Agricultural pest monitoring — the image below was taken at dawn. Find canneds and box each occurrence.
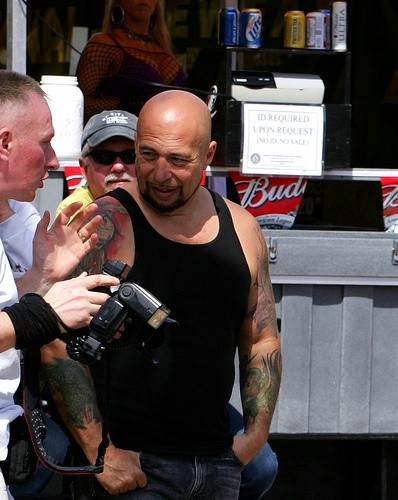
[240,8,262,48]
[282,10,327,50]
[217,7,240,46]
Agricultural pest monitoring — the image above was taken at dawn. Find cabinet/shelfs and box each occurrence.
[220,43,352,171]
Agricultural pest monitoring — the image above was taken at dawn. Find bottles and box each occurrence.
[38,75,84,160]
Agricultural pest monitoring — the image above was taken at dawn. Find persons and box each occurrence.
[75,1,187,124]
[0,70,120,500]
[25,110,280,500]
[46,90,283,500]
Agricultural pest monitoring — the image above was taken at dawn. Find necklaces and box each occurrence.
[119,23,156,43]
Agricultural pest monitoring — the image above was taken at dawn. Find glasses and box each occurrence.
[84,149,136,165]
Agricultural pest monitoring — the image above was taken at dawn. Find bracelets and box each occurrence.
[3,292,61,351]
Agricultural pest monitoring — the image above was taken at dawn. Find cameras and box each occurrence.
[66,259,170,363]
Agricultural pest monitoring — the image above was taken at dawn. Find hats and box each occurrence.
[81,109,138,150]
